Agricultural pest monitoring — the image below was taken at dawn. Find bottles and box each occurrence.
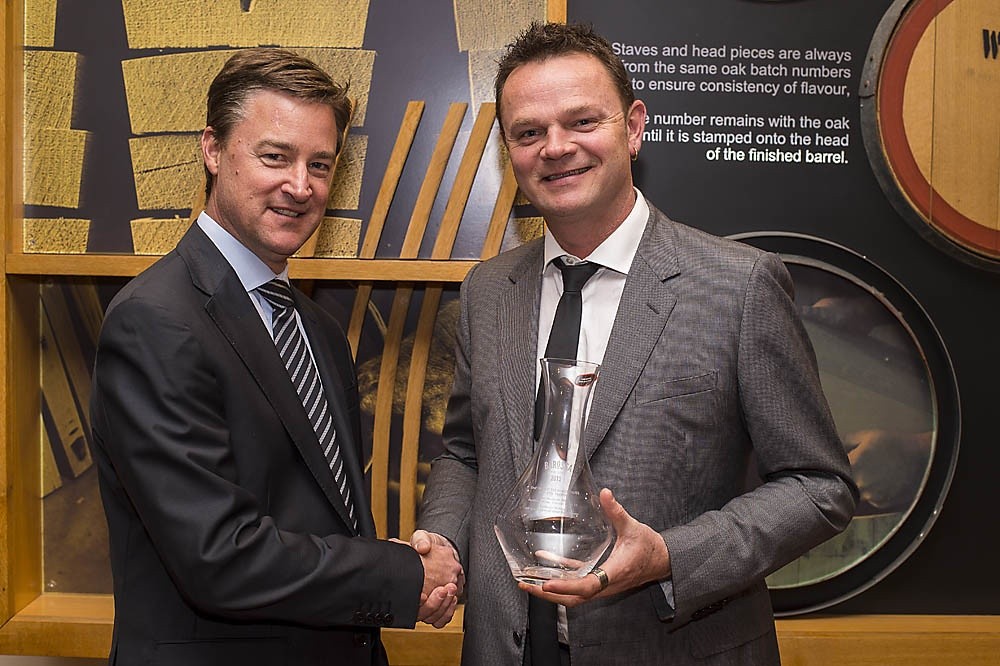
[493,358,613,589]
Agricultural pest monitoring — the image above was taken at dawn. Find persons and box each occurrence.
[90,45,464,666]
[411,20,865,666]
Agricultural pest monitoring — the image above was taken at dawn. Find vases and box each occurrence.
[493,357,613,586]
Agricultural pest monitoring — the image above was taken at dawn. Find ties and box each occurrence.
[255,279,360,539]
[526,256,602,666]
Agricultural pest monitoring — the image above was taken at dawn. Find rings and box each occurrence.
[591,569,609,591]
